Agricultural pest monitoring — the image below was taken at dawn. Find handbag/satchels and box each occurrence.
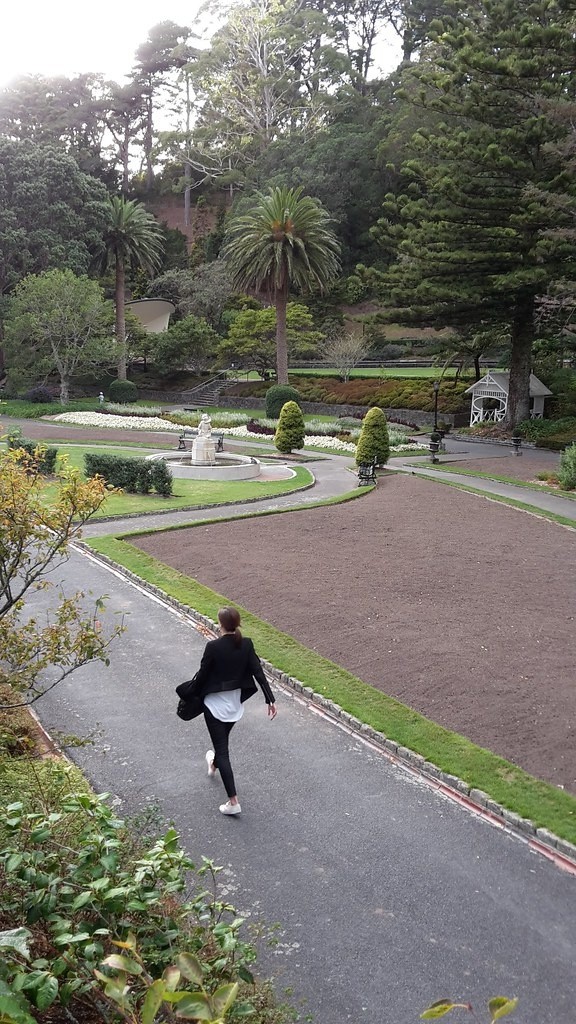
[177,671,203,721]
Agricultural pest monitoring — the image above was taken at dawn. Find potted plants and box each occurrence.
[428,433,441,449]
[510,428,523,443]
[435,421,445,436]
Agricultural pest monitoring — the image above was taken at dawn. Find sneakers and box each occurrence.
[219,801,241,814]
[206,750,216,777]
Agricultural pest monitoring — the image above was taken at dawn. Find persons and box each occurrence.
[218,436,223,451]
[197,606,277,815]
[198,414,212,440]
[98,392,104,404]
[179,434,185,447]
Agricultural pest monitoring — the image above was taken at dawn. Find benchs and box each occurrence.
[178,428,224,452]
[358,456,379,488]
